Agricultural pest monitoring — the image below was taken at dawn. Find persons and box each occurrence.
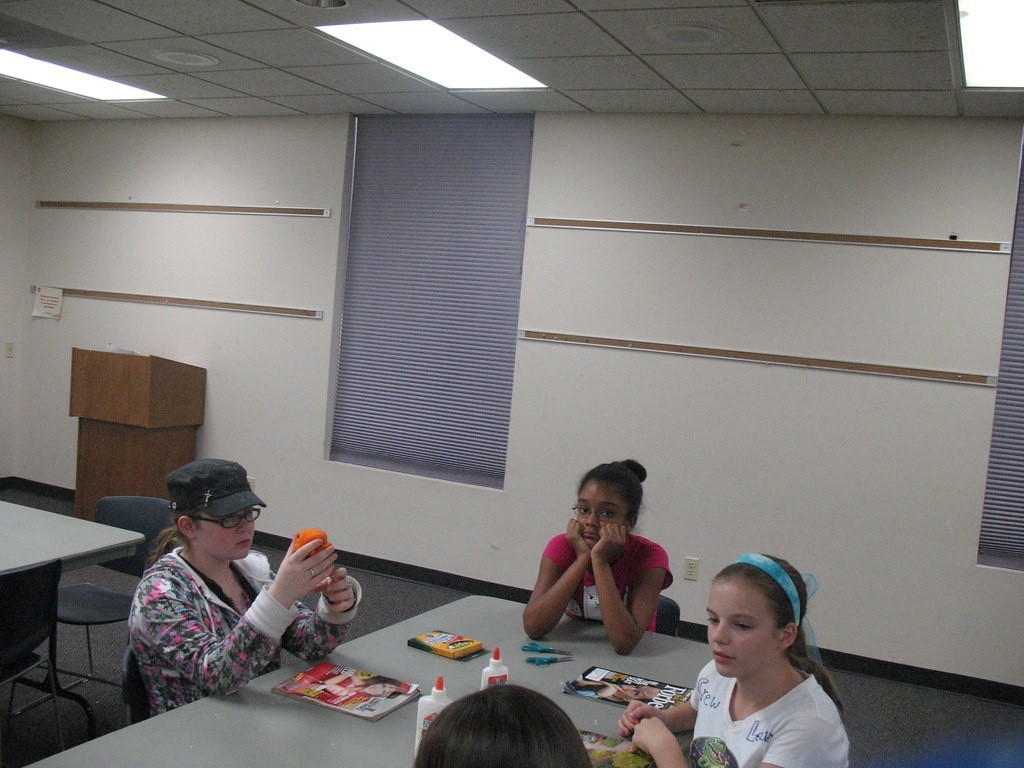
[523,459,673,656]
[412,686,593,768]
[128,459,363,718]
[617,553,849,768]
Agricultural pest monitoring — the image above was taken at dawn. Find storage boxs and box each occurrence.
[407,630,483,659]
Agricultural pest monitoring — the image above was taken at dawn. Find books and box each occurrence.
[271,660,422,722]
[562,665,694,711]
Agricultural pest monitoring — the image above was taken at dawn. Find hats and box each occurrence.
[166,458,267,516]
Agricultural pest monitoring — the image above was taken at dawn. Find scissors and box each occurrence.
[521,642,572,654]
[526,656,572,666]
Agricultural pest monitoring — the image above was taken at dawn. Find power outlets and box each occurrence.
[247,476,256,491]
[684,557,700,581]
[4,342,14,358]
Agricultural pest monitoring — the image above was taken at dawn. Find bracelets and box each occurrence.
[323,588,356,613]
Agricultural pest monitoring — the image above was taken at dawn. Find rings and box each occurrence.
[310,569,315,576]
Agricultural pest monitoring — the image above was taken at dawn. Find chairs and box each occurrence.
[0,559,63,754]
[54,495,172,683]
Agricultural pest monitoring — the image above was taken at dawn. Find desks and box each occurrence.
[0,500,145,741]
[19,595,715,768]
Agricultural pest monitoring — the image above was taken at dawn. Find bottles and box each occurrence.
[479,646,508,690]
[413,675,455,759]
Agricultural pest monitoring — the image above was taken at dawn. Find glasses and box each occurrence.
[571,506,629,522]
[186,508,261,528]
